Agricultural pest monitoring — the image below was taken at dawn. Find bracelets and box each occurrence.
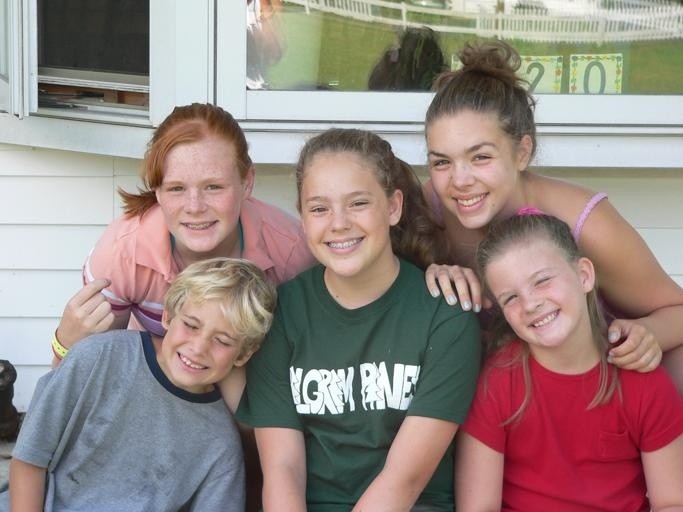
[50,344,63,363]
[51,326,68,359]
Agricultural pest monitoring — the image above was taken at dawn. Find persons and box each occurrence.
[231,125,487,512]
[0,255,280,511]
[453,205,682,512]
[50,101,319,511]
[417,33,682,396]
[363,23,445,93]
